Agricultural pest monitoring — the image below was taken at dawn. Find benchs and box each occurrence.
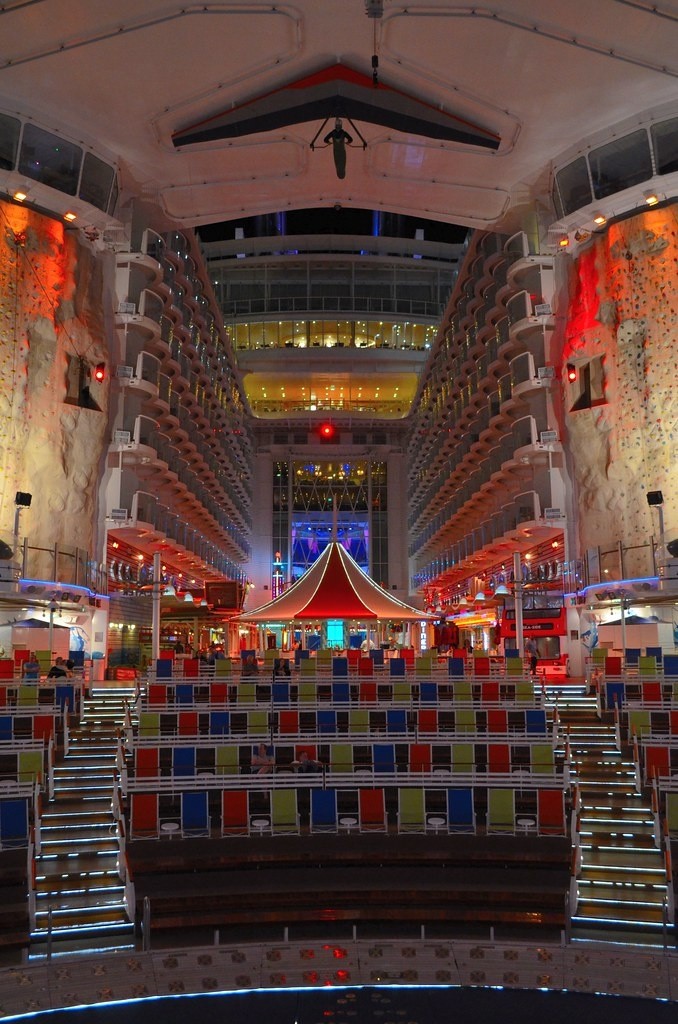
[0,835,571,956]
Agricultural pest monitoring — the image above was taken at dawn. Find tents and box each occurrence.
[224,542,444,650]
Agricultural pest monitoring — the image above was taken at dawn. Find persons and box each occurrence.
[525,634,541,674]
[251,744,276,799]
[447,639,474,656]
[23,654,41,686]
[275,658,291,676]
[239,633,249,661]
[361,636,376,651]
[47,657,74,679]
[292,639,302,651]
[140,654,150,666]
[243,655,260,676]
[291,752,323,773]
[175,640,226,678]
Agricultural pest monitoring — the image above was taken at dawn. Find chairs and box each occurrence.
[0,646,678,836]
